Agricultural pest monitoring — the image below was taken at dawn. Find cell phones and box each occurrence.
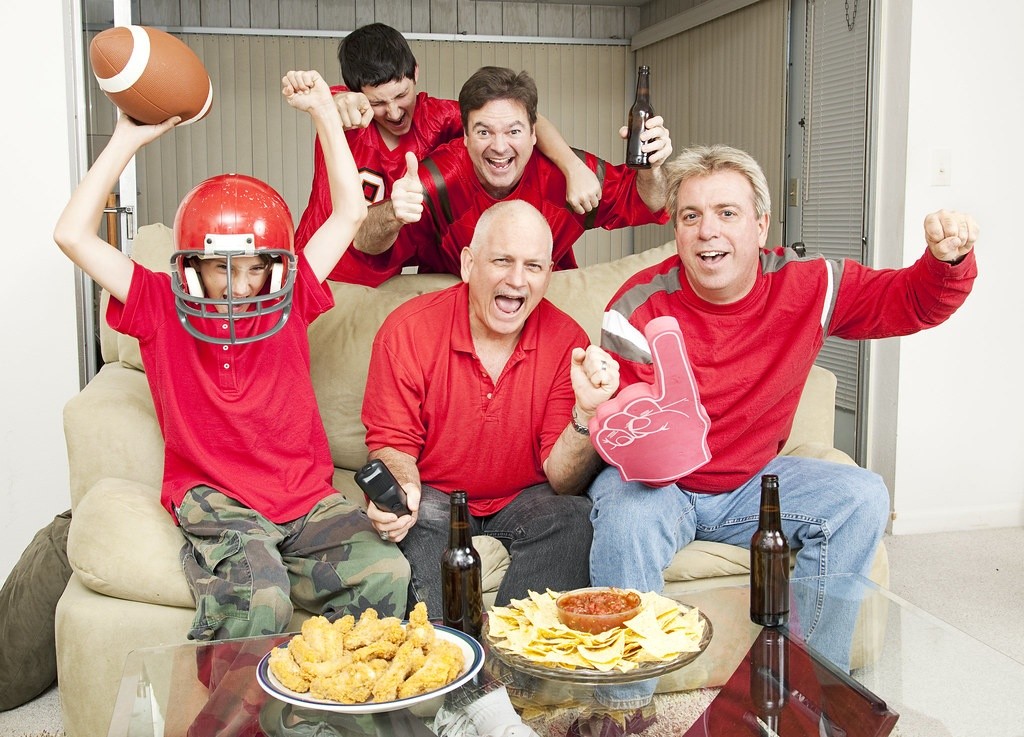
[354,458,412,517]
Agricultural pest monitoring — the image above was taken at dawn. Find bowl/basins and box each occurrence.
[556,587,643,636]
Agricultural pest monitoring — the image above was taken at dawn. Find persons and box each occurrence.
[281,23,602,288]
[563,143,977,737]
[51,64,416,736]
[358,198,622,737]
[352,55,674,285]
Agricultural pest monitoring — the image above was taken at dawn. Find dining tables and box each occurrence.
[111,570,1024,737]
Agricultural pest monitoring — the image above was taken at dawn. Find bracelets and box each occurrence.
[569,404,594,437]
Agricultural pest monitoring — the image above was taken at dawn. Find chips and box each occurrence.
[487,587,705,671]
[507,692,663,729]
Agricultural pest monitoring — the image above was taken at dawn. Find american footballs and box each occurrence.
[89,24,214,128]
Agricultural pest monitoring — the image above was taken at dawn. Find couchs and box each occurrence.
[54,222,889,737]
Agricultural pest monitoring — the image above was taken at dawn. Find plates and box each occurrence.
[256,618,486,715]
[480,590,715,685]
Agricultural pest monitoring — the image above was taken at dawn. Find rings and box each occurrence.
[379,531,389,541]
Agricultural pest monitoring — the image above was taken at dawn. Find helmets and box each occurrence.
[176,173,296,296]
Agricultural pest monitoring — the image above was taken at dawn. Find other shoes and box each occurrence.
[566,696,656,737]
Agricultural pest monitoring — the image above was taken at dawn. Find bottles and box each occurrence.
[625,65,655,169]
[749,474,791,627]
[749,625,789,737]
[441,490,484,646]
